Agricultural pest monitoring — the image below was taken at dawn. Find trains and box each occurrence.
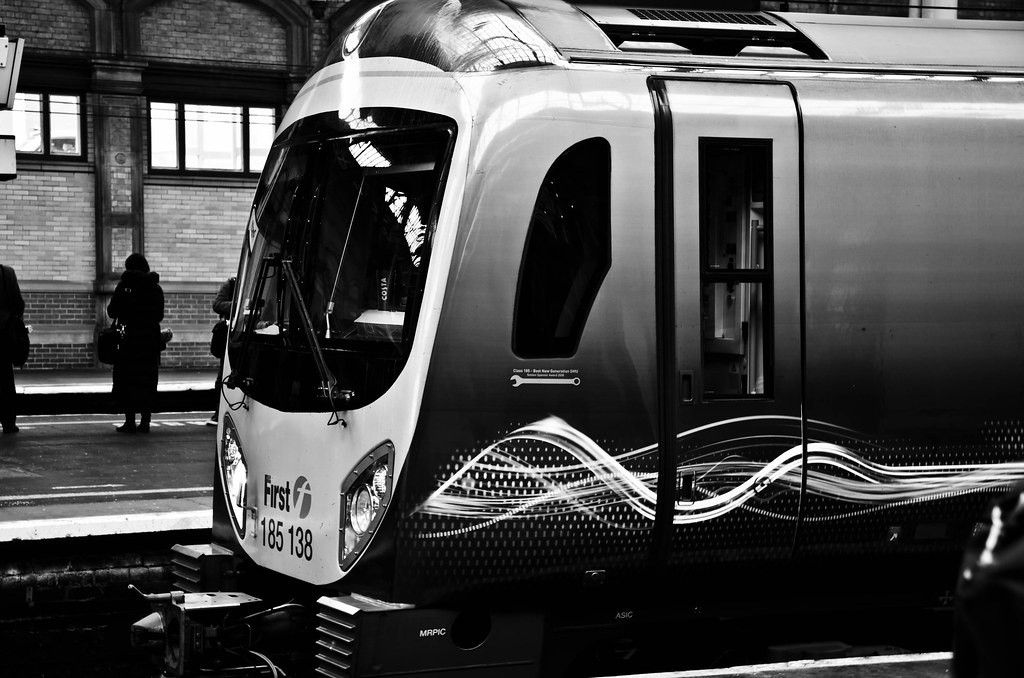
[127,1,1024,678]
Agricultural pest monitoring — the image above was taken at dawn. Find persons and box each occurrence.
[0,263,30,436]
[206,276,237,425]
[106,253,164,431]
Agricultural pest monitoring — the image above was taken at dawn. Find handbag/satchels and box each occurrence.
[210,320,228,357]
[98,317,123,365]
[5,317,30,366]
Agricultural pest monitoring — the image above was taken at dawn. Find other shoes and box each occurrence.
[116,420,136,433]
[3,424,19,434]
[207,413,218,426]
[137,420,149,432]
